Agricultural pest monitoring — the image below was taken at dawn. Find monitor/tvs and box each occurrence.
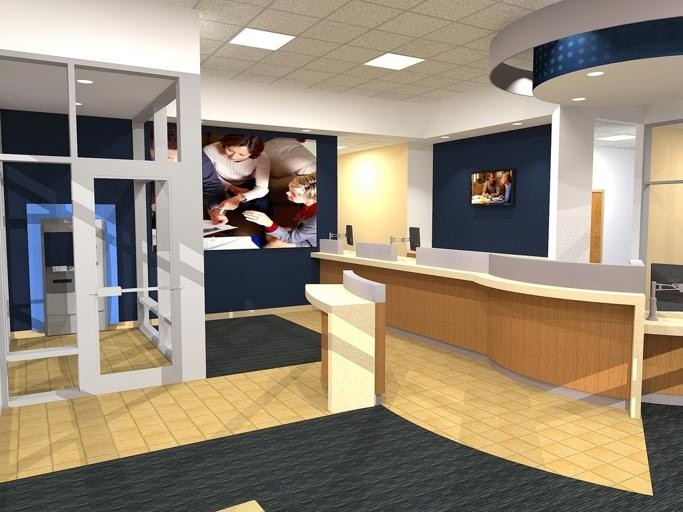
[410,227,420,251]
[470,169,515,206]
[347,225,353,245]
[651,263,683,311]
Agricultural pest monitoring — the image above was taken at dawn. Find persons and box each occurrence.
[242,172,318,249]
[203,133,272,233]
[149,127,229,226]
[490,172,512,203]
[482,172,500,198]
